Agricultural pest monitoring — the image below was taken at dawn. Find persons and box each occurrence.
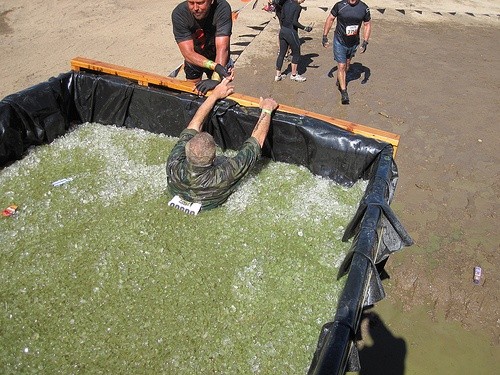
[322,0,372,104]
[165,76,279,216]
[261,0,315,82]
[171,0,235,95]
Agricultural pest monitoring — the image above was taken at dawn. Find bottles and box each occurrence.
[473,266,481,284]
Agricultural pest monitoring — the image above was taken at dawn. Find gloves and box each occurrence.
[304,26,312,32]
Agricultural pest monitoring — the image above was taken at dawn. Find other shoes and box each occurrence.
[341,89,349,103]
[290,73,307,82]
[336,78,347,92]
[274,74,286,82]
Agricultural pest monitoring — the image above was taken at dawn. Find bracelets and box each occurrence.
[206,60,213,69]
[262,108,272,115]
